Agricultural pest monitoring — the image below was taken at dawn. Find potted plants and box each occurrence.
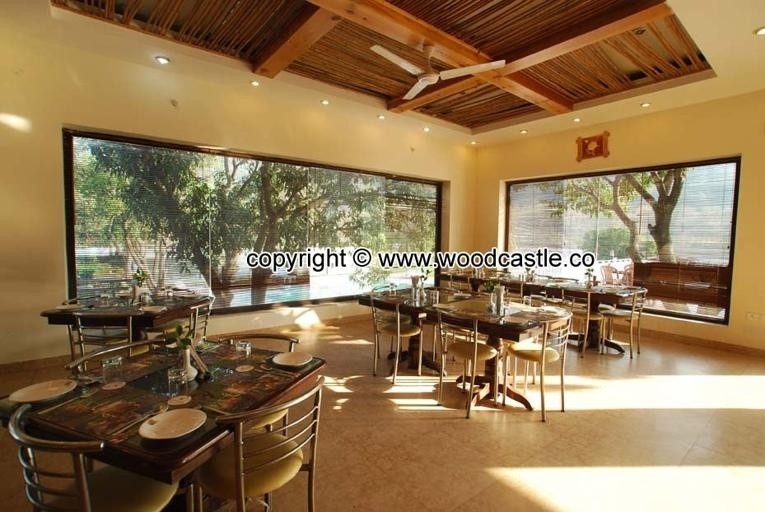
[130,266,151,304]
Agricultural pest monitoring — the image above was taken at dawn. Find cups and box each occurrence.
[523,295,531,312]
[235,342,251,370]
[100,355,124,390]
[430,290,438,306]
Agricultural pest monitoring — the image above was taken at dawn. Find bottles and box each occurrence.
[168,367,188,406]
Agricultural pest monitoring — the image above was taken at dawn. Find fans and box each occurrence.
[368,43,506,102]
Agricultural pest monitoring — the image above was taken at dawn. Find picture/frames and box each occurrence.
[576,131,610,162]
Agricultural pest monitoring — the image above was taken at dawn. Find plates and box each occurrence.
[138,306,167,314]
[272,351,313,367]
[8,378,77,404]
[55,304,86,312]
[138,409,208,440]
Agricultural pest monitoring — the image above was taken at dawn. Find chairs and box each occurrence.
[359,261,647,413]
[0,286,327,512]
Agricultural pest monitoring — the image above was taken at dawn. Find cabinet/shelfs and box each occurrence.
[717,267,730,309]
[632,263,679,298]
[679,264,717,304]
